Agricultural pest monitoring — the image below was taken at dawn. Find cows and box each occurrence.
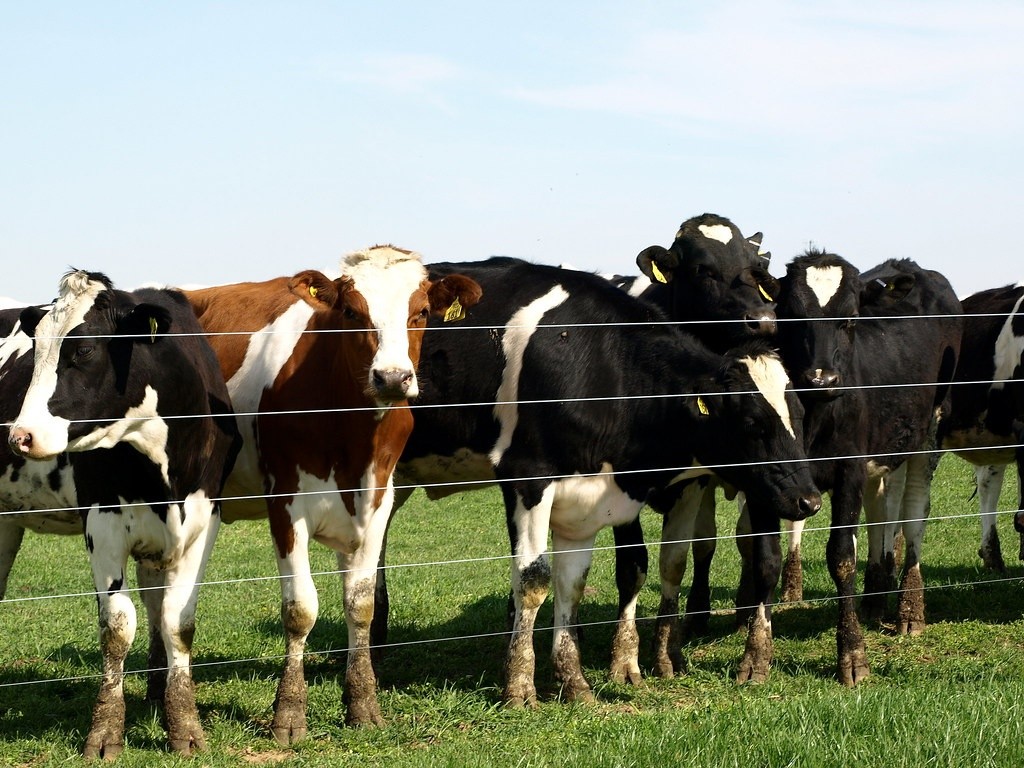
[0,213,1024,762]
